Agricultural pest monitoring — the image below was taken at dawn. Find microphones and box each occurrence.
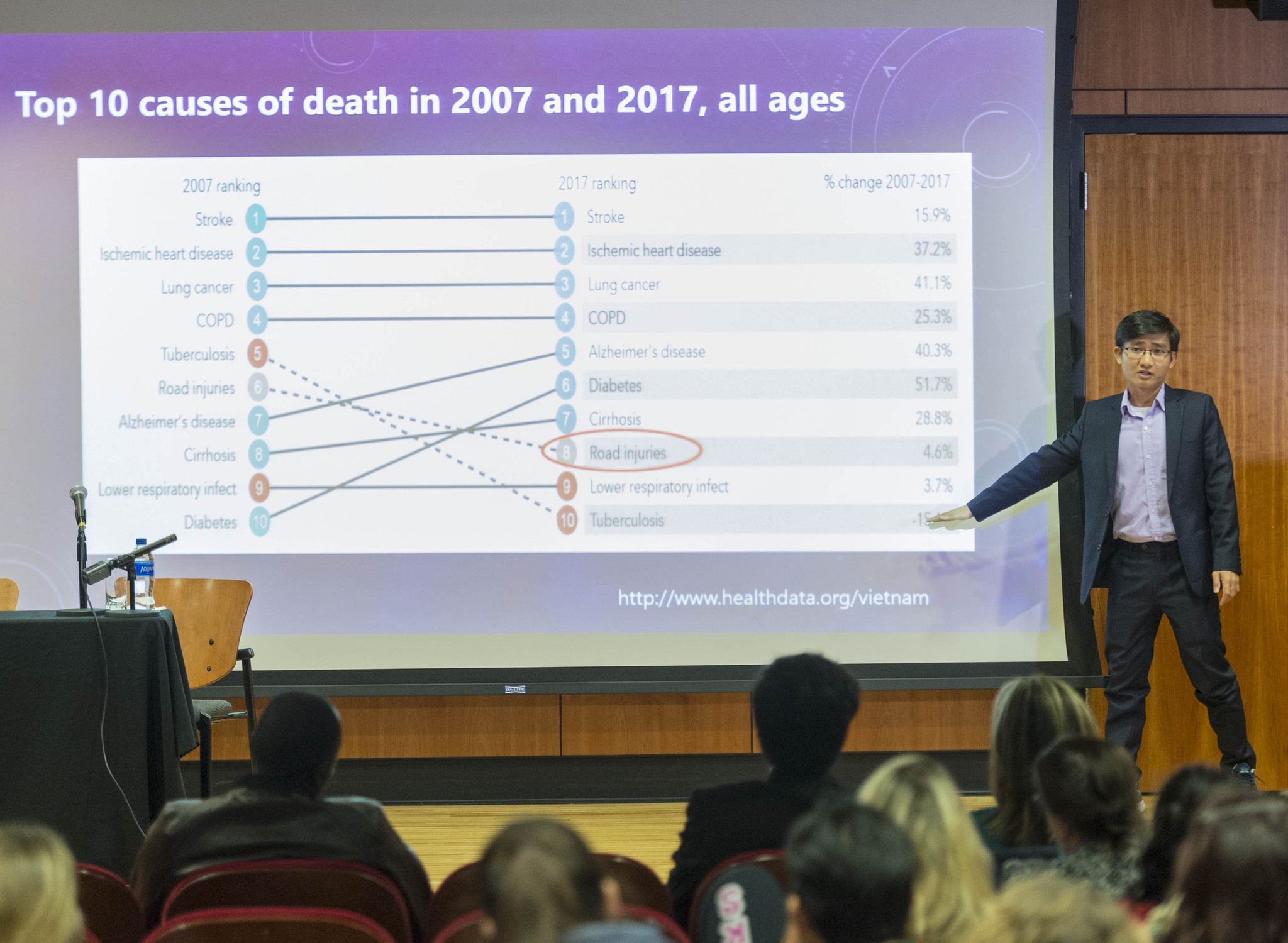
[82,534,177,586]
[70,485,87,532]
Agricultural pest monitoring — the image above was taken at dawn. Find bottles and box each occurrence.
[128,538,156,610]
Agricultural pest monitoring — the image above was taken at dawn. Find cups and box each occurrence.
[104,558,127,611]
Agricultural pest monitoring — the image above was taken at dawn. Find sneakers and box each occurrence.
[1230,763,1265,791]
[1135,783,1146,814]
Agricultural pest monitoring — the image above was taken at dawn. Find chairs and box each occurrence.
[114,578,257,800]
[71,853,794,943]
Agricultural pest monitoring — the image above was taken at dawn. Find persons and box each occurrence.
[0,652,1288,943]
[926,310,1256,815]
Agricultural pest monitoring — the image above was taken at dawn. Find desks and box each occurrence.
[0,607,202,894]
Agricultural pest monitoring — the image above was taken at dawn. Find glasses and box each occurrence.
[1120,346,1172,358]
